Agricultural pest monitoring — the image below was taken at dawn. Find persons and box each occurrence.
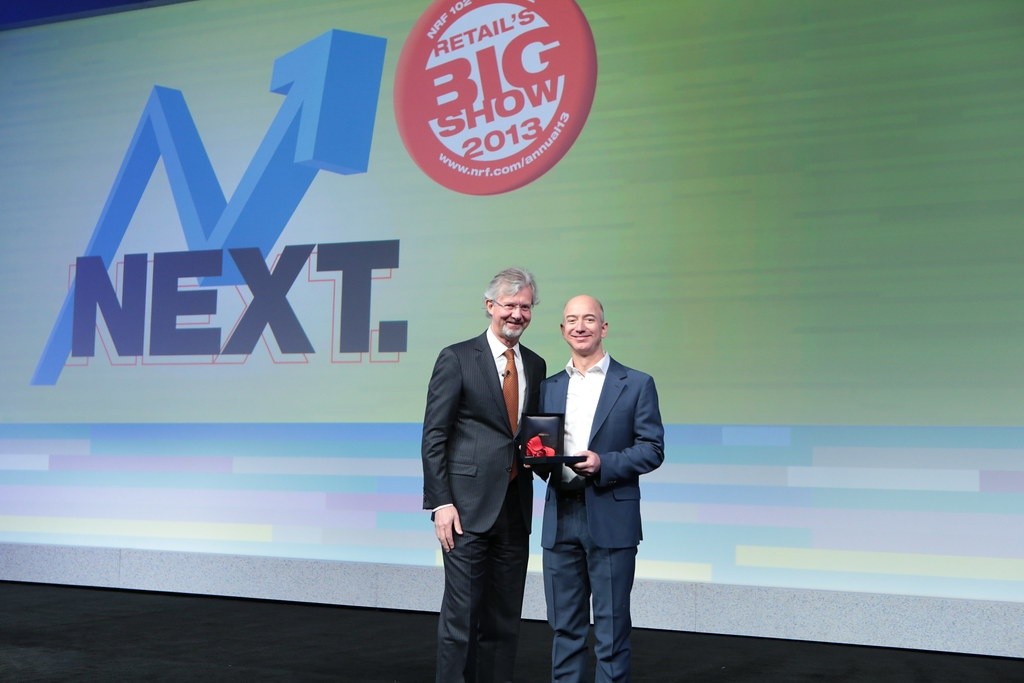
[518,294,665,683]
[421,267,547,683]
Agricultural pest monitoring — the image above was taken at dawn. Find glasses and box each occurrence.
[493,300,532,313]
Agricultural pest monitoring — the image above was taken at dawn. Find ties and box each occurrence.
[503,349,519,482]
